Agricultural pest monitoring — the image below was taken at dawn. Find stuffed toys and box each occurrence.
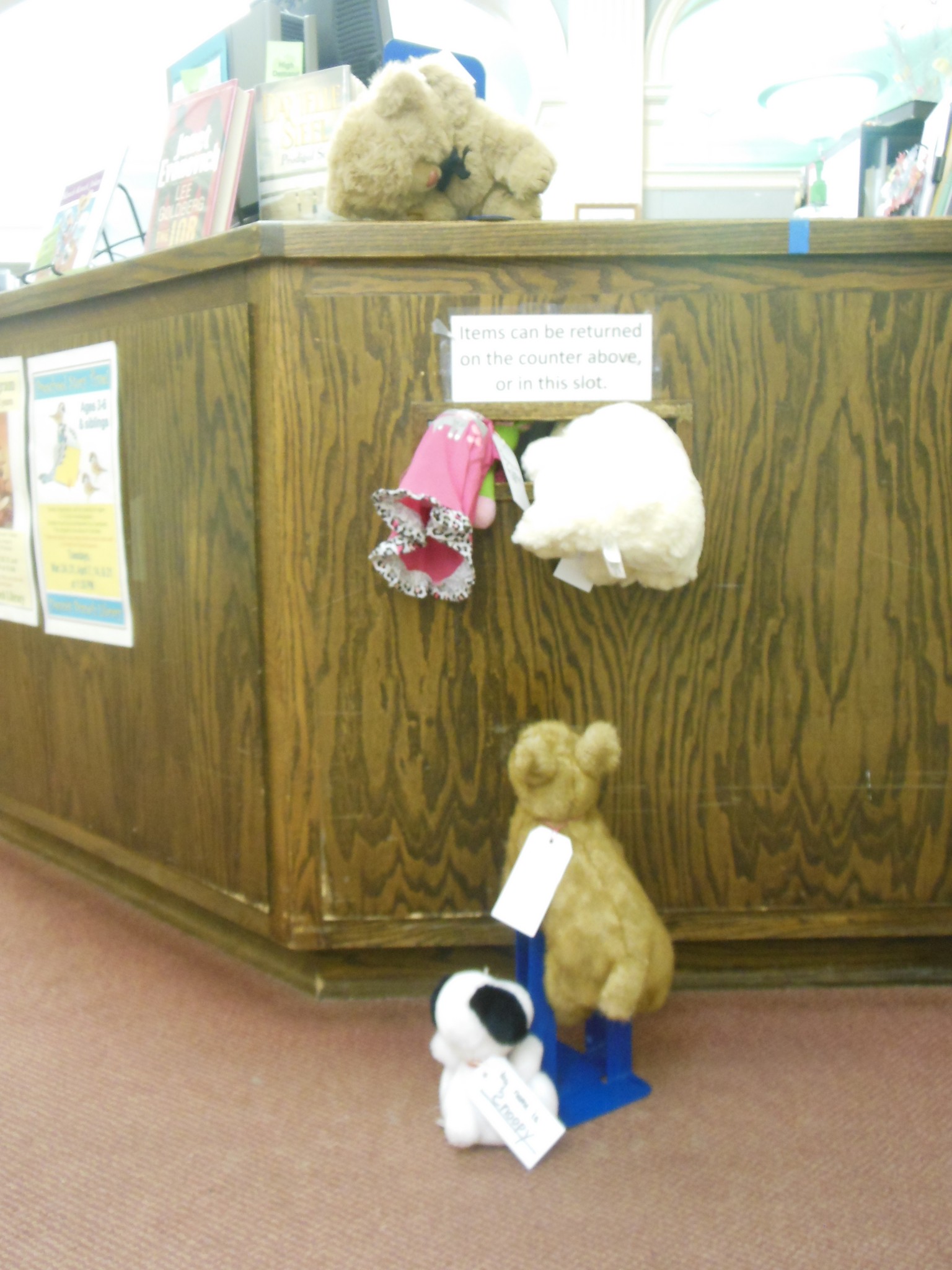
[428,967,559,1149]
[500,718,675,1028]
[326,48,556,222]
[366,400,706,604]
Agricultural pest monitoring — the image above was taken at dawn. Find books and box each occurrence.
[24,166,109,284]
[252,64,351,222]
[141,79,253,252]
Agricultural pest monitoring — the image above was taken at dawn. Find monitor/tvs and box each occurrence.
[164,0,393,225]
